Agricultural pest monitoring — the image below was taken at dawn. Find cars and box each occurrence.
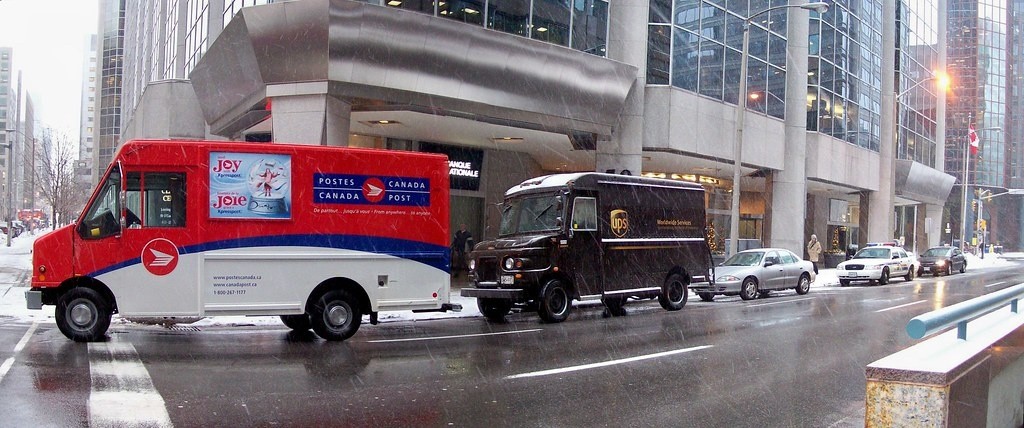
[837,242,915,287]
[0,216,48,240]
[917,246,968,277]
[690,247,816,301]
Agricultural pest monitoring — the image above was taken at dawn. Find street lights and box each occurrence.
[960,126,1002,251]
[729,2,830,256]
[889,70,951,248]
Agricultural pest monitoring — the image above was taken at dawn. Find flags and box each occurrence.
[969,123,980,154]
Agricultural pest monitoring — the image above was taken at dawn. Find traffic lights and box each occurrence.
[988,190,993,203]
[972,201,977,212]
[974,190,979,197]
[982,220,986,228]
[974,219,979,231]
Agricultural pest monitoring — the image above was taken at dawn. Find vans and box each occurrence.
[23,140,463,342]
[461,173,715,323]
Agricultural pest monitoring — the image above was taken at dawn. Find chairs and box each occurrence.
[156,184,181,226]
[579,220,595,229]
[870,250,877,256]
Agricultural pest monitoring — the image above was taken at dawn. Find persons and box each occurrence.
[807,234,821,275]
[454,223,474,279]
